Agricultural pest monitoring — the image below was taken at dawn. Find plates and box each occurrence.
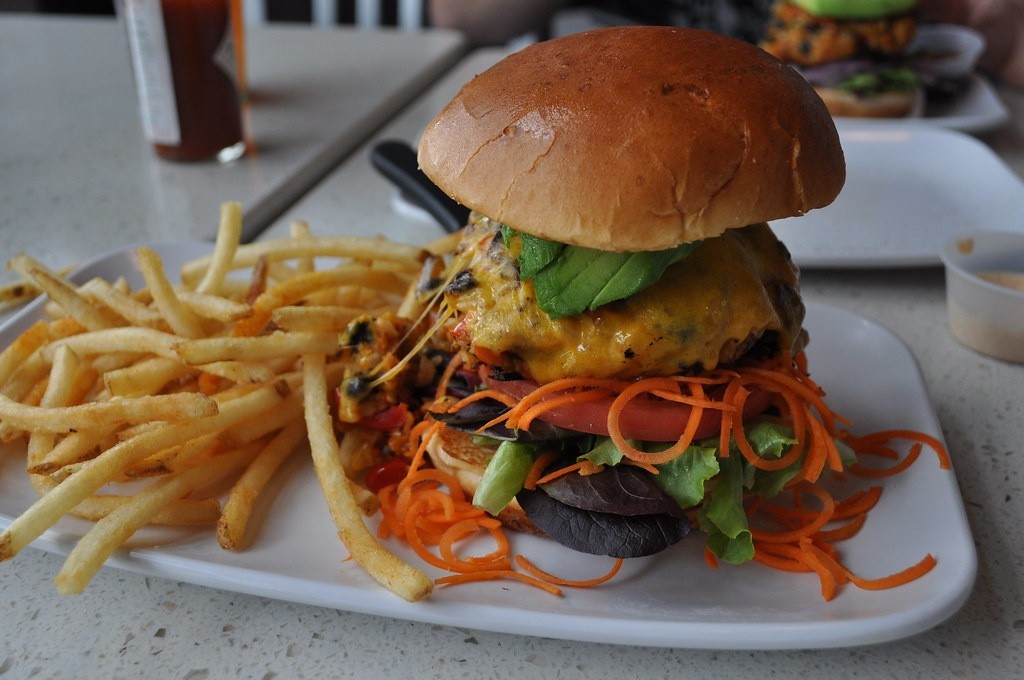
[767,126,1024,271]
[832,71,1009,135]
[1,239,979,651]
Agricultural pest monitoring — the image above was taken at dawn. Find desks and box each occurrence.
[0,13,1024,680]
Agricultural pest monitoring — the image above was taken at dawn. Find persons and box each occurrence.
[426,0,777,52]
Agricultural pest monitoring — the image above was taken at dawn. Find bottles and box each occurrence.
[114,0,257,164]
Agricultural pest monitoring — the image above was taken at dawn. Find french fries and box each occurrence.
[0,200,467,604]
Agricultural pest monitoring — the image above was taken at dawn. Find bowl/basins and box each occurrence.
[935,230,1024,364]
[903,25,985,79]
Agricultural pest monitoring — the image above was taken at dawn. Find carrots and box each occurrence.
[690,429,954,598]
[371,471,623,596]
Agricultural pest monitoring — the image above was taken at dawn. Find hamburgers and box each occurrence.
[340,25,848,561]
[752,1,932,120]
